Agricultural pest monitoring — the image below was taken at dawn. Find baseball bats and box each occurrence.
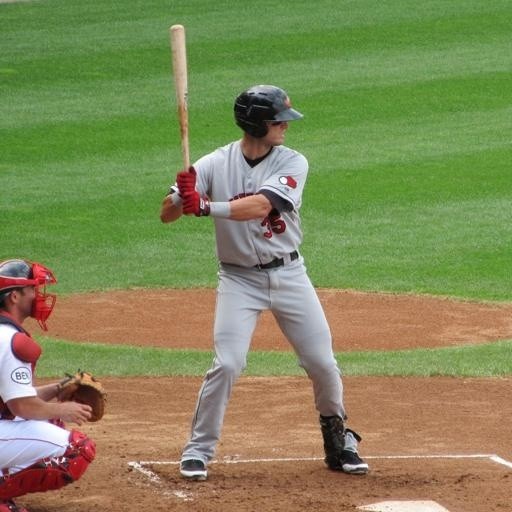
[170,24,192,215]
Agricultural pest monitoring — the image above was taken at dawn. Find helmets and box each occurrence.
[0,259,56,331]
[234,85,304,138]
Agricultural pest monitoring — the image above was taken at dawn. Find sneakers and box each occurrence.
[326,444,370,474]
[179,459,208,481]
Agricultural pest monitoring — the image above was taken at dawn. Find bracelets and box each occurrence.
[210,201,231,219]
[171,189,185,208]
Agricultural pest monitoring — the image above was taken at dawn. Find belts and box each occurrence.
[256,250,298,271]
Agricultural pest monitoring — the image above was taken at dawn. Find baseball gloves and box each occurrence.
[57,371,106,422]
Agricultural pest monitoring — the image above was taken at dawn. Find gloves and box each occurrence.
[175,165,210,217]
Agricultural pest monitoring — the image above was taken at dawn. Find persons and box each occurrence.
[160,84,369,483]
[0,258,96,512]
